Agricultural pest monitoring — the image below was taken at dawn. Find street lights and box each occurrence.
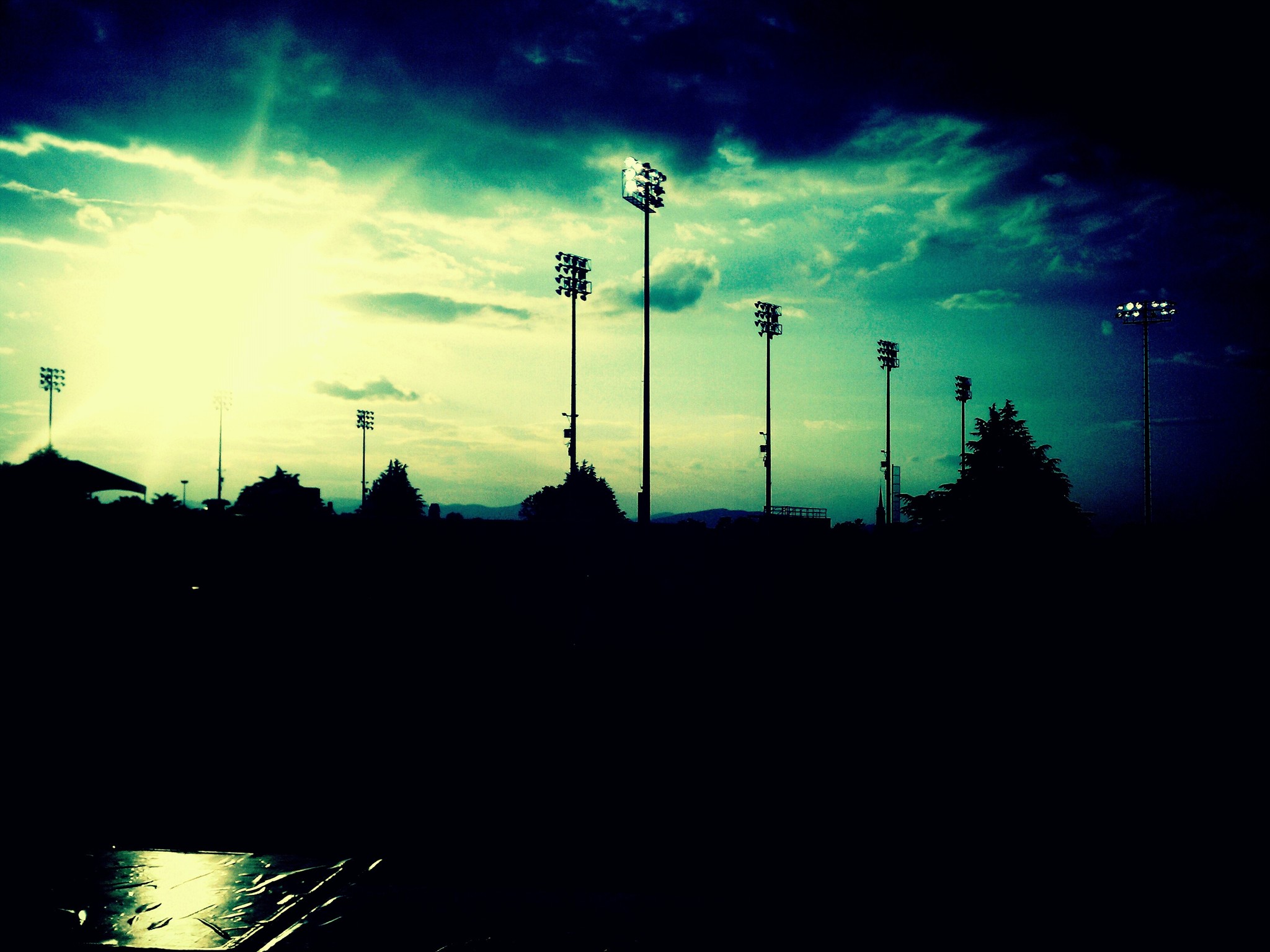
[356,410,374,507]
[555,252,592,475]
[755,301,783,512]
[620,156,665,529]
[873,341,899,523]
[955,375,972,475]
[39,366,65,448]
[1115,296,1180,522]
